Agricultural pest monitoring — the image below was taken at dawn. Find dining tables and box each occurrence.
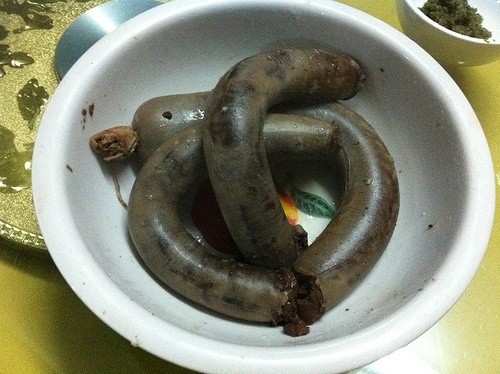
[0,1,500,373]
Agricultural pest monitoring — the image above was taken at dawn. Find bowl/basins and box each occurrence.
[396,0,499,72]
[32,0,495,374]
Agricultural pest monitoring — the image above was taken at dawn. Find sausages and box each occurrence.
[93,46,404,338]
